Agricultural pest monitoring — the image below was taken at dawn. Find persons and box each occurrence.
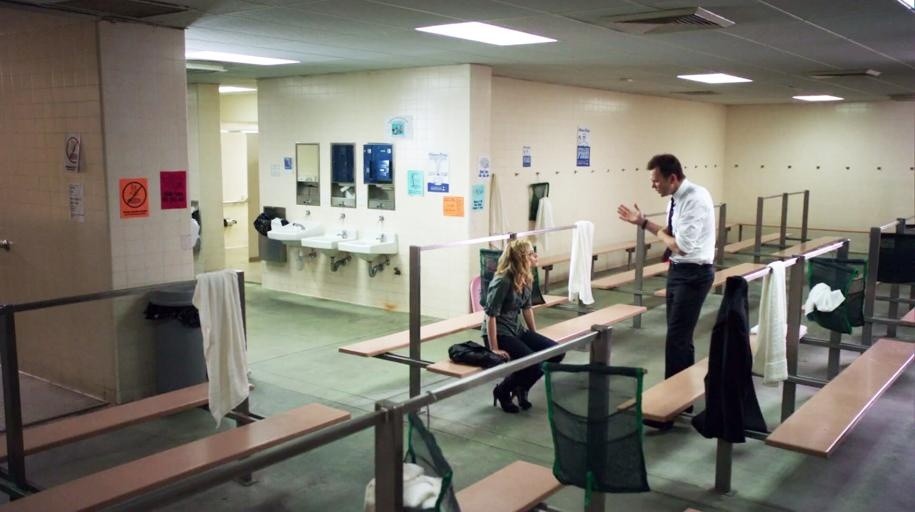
[616,155,716,430]
[481,239,566,413]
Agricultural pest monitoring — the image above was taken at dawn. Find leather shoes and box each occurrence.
[642,419,674,431]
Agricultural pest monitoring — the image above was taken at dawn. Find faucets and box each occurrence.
[375,232,384,243]
[337,229,345,238]
[292,222,305,230]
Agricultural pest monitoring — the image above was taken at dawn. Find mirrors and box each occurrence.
[330,142,357,208]
[363,143,395,210]
[295,143,320,207]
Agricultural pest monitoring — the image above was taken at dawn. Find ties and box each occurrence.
[667,197,674,237]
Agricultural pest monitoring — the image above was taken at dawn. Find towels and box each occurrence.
[534,196,561,249]
[489,172,505,251]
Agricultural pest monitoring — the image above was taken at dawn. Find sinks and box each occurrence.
[267,223,323,240]
[301,230,357,250]
[339,229,398,254]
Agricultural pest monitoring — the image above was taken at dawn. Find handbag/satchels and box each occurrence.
[450,341,501,368]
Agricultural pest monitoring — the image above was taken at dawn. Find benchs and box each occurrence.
[0,374,251,463]
[716,233,790,254]
[592,259,673,290]
[428,301,647,380]
[618,322,807,422]
[763,338,915,458]
[770,236,845,259]
[338,294,567,358]
[902,308,915,327]
[3,403,349,511]
[533,239,662,270]
[455,462,568,510]
[654,263,768,296]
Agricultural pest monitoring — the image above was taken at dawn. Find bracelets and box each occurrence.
[641,218,648,231]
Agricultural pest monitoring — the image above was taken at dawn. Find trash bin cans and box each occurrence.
[148,282,207,395]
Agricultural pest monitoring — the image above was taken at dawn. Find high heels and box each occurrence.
[510,384,532,409]
[493,384,519,413]
